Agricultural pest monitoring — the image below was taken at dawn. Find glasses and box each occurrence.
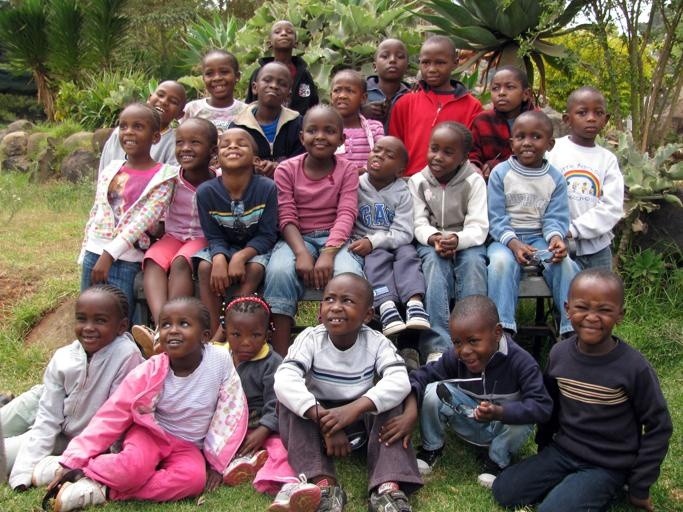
[437,378,497,420]
[230,199,246,241]
[315,396,370,451]
[41,469,84,511]
[523,247,559,266]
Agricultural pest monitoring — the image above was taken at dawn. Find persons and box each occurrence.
[77,100,180,322]
[219,293,322,512]
[99,19,320,178]
[542,86,625,270]
[261,104,365,359]
[378,295,554,491]
[486,109,582,342]
[490,267,674,512]
[196,127,277,342]
[1,283,147,494]
[408,121,491,369]
[330,34,542,184]
[45,296,249,512]
[273,271,425,512]
[131,117,219,359]
[347,135,434,336]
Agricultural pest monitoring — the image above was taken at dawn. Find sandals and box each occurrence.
[132,324,162,358]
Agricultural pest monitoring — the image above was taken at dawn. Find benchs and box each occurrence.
[133,249,565,363]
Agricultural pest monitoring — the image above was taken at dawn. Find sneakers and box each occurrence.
[379,307,406,337]
[477,451,514,488]
[368,490,413,512]
[32,456,63,488]
[223,449,269,485]
[416,441,446,474]
[54,476,108,512]
[266,474,346,511]
[406,304,431,330]
